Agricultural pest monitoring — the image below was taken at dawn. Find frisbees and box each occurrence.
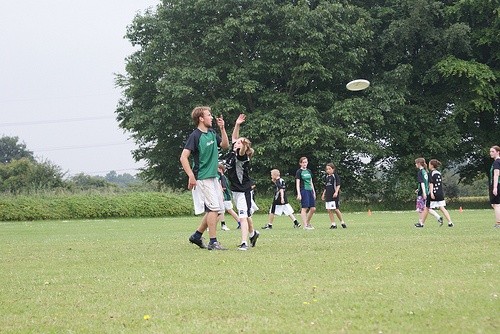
[346,79,370,91]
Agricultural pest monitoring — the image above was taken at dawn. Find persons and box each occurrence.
[206,161,256,231]
[489,146,500,228]
[415,159,454,227]
[414,158,443,226]
[321,163,346,229]
[230,114,260,250]
[180,107,229,250]
[261,169,301,229]
[295,157,316,230]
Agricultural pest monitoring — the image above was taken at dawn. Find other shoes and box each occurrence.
[261,224,273,230]
[236,222,241,229]
[414,222,424,228]
[293,223,301,228]
[237,243,250,251]
[447,223,455,228]
[188,235,207,248]
[207,241,228,250]
[330,224,338,229]
[438,216,444,227]
[303,223,315,230]
[221,225,230,231]
[249,230,260,247]
[341,221,347,228]
